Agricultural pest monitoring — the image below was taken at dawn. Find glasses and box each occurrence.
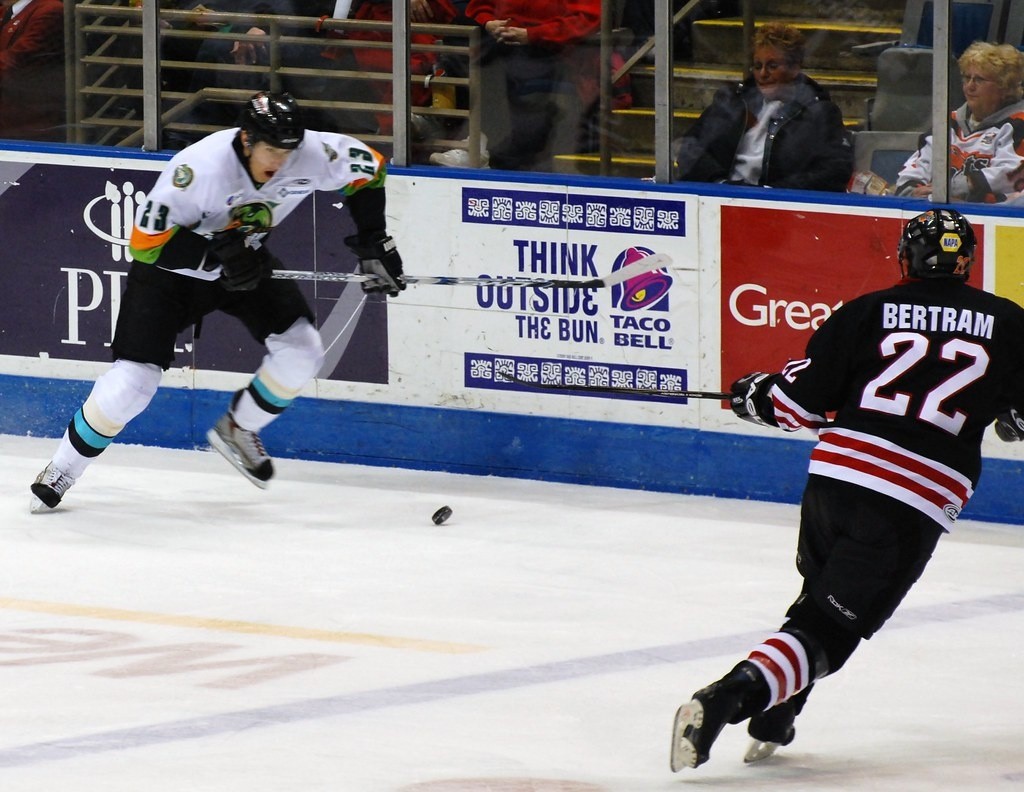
[961,71,995,87]
[748,60,790,74]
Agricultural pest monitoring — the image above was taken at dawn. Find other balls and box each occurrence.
[431,505,453,526]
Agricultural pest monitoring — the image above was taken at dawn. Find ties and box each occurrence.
[0,6,13,31]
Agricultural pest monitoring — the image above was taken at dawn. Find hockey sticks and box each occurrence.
[496,369,731,403]
[268,252,679,289]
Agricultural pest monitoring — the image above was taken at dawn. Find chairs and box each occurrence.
[862,44,962,135]
[848,131,926,184]
[853,0,1007,59]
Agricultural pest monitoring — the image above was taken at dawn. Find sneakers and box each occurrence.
[670,659,771,772]
[429,139,491,170]
[743,684,814,762]
[29,460,75,514]
[206,388,274,489]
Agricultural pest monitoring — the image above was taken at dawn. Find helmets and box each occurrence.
[241,91,305,149]
[898,208,977,282]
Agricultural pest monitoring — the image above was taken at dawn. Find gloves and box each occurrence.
[202,225,273,291]
[730,372,780,428]
[344,233,407,298]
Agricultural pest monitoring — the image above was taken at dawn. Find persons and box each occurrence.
[672,208,1024,777]
[0,0,1024,206]
[31,90,406,512]
